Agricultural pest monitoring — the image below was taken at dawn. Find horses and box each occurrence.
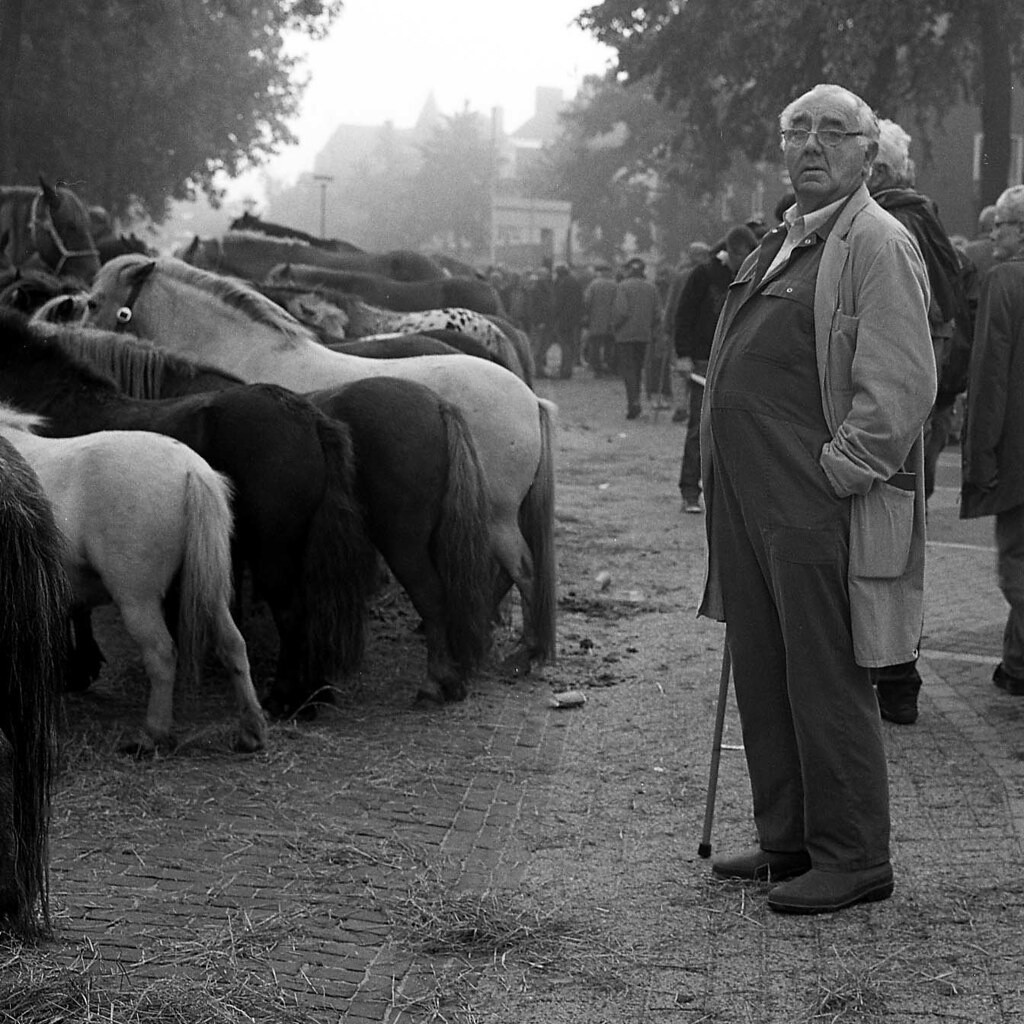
[0,171,559,943]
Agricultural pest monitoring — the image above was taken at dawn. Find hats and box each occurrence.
[622,258,647,271]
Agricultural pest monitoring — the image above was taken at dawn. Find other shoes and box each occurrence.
[993,665,1024,696]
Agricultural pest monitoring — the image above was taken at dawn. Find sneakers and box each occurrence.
[875,683,922,723]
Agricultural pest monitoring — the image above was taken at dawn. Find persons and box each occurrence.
[698,84,944,916]
[957,183,1024,698]
[484,203,1020,420]
[858,113,970,724]
[675,224,766,514]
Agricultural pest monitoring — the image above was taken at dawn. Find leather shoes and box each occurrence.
[765,859,896,916]
[710,845,811,878]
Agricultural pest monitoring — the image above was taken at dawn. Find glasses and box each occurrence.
[782,126,864,146]
[990,216,1020,231]
